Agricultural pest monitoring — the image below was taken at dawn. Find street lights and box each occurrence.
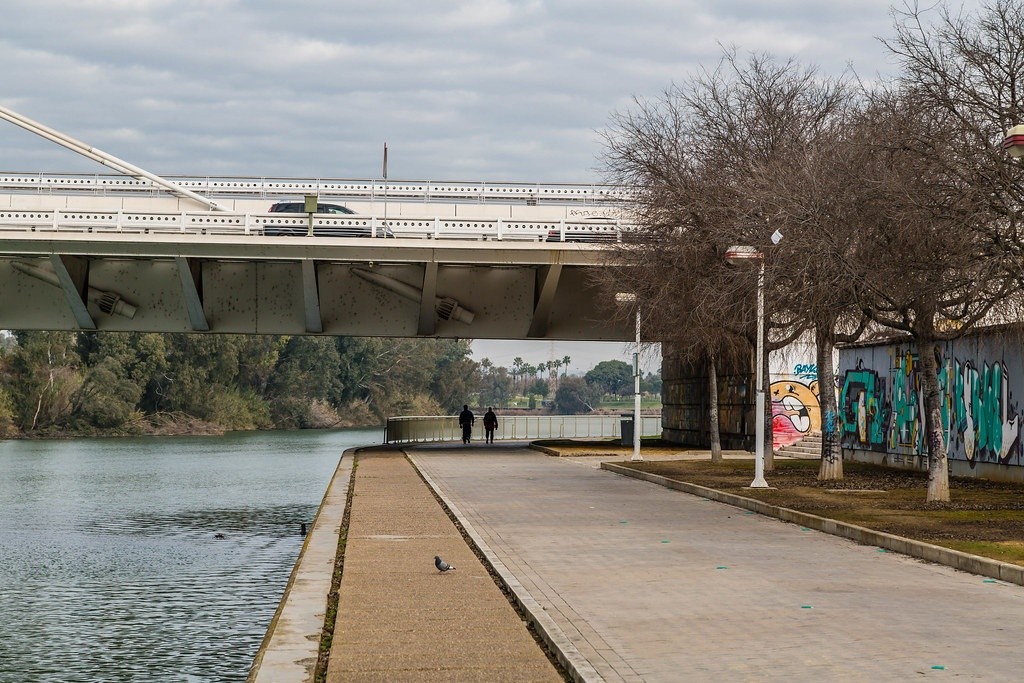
[723,244,777,489]
[614,292,645,461]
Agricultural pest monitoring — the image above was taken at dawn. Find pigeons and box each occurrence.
[433,556,456,573]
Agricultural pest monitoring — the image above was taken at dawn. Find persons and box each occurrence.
[459,404,474,444]
[483,407,498,444]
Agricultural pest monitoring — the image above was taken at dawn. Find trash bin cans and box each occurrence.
[620,414,634,446]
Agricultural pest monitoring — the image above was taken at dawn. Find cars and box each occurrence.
[257,203,398,241]
